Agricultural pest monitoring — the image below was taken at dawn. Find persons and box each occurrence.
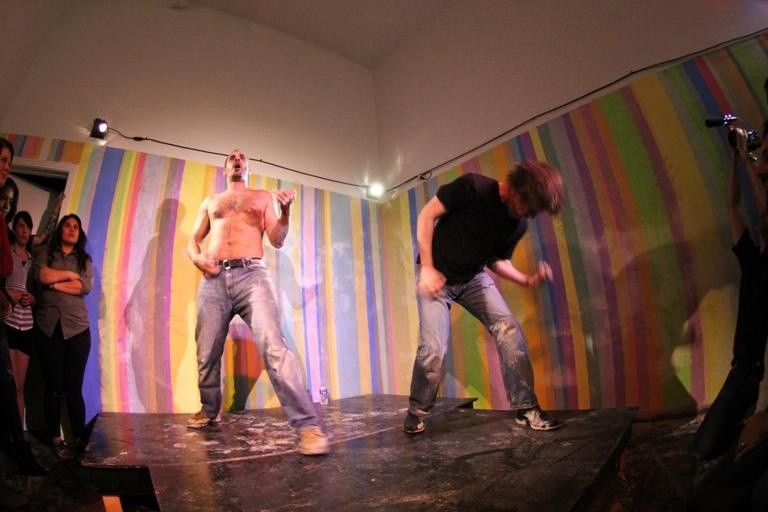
[183,147,331,458]
[688,125,768,512]
[33,211,95,463]
[399,157,567,436]
[1,137,51,477]
[0,177,66,250]
[3,210,37,428]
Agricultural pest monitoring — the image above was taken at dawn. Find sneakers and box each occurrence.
[297,427,330,454]
[515,406,563,431]
[186,407,221,429]
[403,408,426,434]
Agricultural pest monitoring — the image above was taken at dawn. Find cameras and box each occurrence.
[728,128,760,153]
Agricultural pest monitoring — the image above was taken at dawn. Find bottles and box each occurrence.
[320,384,329,404]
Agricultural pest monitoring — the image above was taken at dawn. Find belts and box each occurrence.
[217,258,252,271]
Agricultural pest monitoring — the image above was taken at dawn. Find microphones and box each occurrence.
[704,115,741,128]
